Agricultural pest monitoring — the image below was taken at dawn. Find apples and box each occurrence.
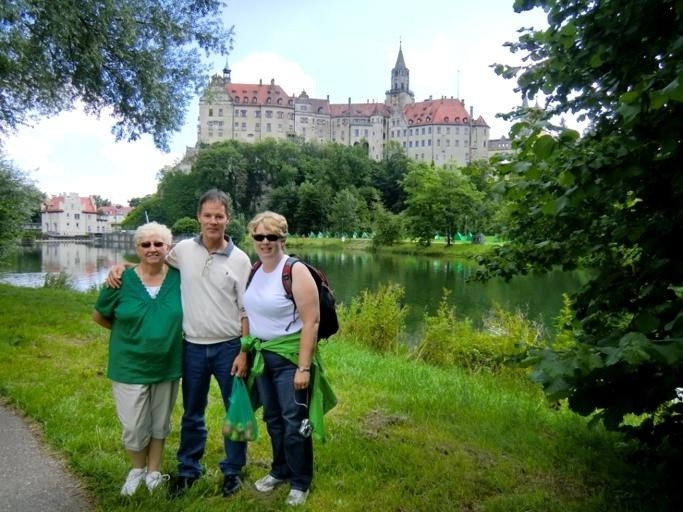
[221,420,254,442]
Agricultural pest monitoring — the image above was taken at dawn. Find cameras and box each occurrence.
[299,418,314,438]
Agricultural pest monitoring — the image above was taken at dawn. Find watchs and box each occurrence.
[296,366,310,372]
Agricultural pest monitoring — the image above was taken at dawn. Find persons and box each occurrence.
[242,211,322,508]
[90,220,183,498]
[104,189,257,500]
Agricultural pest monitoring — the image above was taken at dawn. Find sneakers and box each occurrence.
[120,465,309,508]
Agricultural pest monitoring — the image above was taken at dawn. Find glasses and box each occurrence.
[136,242,166,247]
[252,234,282,241]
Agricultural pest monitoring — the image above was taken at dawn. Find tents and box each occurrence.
[286,230,473,242]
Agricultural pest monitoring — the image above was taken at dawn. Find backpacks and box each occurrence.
[245,253,339,339]
